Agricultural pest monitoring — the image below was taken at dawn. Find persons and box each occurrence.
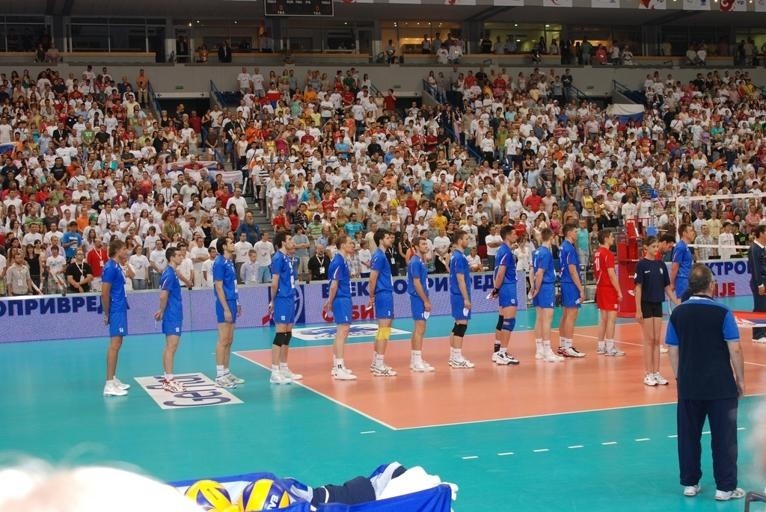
[665,262,746,500]
[0,32,766,397]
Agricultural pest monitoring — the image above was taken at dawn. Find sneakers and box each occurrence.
[604,343,626,356]
[373,367,398,376]
[214,375,237,389]
[447,356,475,369]
[714,487,746,501]
[223,371,246,384]
[535,348,545,359]
[683,483,701,496]
[643,372,659,386]
[557,345,577,353]
[162,376,185,393]
[751,336,766,344]
[595,344,607,354]
[653,373,669,385]
[280,369,303,379]
[103,381,128,396]
[270,374,294,384]
[369,361,393,371]
[660,344,669,353]
[542,350,564,362]
[331,367,353,375]
[410,360,435,372]
[334,369,357,379]
[562,346,586,357]
[112,376,130,389]
[496,352,520,364]
[491,350,516,361]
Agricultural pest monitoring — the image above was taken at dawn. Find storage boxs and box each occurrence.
[166,472,310,511]
[314,464,452,512]
